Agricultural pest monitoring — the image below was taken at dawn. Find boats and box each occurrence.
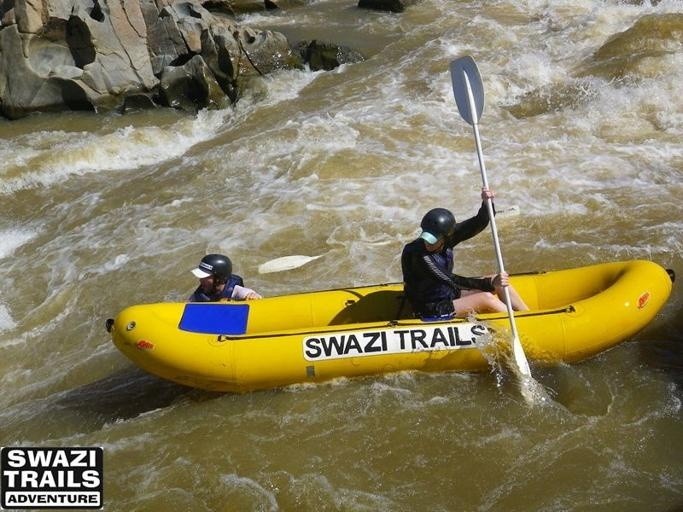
[103,257,674,396]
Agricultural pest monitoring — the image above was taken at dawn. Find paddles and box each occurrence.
[256,240,394,274]
[450,54,533,376]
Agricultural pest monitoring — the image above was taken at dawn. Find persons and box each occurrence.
[181,251,265,305]
[399,186,530,324]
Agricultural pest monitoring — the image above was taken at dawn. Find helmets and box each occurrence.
[421,208,457,237]
[199,254,232,284]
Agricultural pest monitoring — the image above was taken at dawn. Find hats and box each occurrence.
[419,229,445,246]
[190,266,214,280]
